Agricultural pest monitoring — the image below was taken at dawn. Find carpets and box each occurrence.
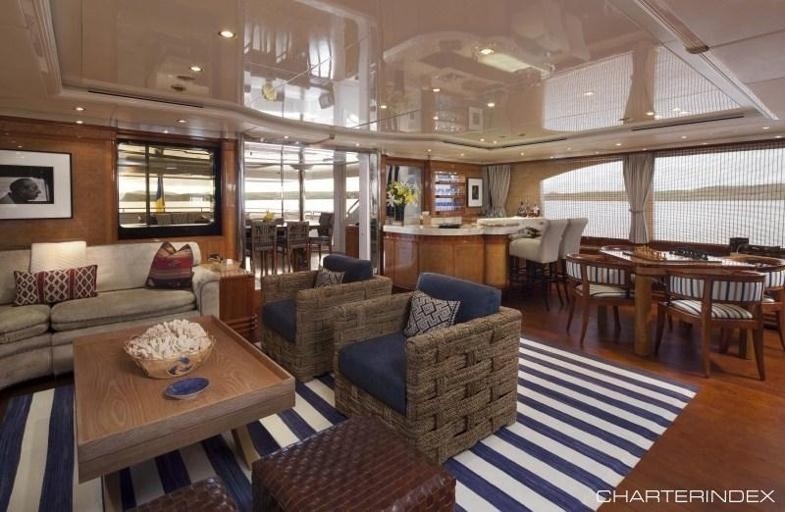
[0,331,701,512]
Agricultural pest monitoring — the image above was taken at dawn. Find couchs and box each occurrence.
[1,240,206,393]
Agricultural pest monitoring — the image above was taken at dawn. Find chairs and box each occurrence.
[257,251,393,386]
[505,207,785,385]
[330,269,524,466]
[241,207,337,277]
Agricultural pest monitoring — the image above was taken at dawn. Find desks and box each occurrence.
[71,315,300,498]
[200,259,259,341]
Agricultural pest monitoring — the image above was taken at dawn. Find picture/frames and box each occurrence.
[0,146,77,222]
[466,176,484,208]
[465,105,486,134]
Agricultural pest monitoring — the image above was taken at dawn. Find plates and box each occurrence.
[164,377,210,400]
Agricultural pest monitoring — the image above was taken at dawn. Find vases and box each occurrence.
[392,198,406,226]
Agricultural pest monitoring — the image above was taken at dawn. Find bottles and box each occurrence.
[419,215,424,230]
[433,170,466,211]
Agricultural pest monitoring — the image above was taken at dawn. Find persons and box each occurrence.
[0,178,41,203]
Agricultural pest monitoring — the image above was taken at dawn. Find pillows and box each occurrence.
[11,261,100,308]
[143,240,195,293]
[30,239,88,276]
[402,289,464,336]
[314,263,347,288]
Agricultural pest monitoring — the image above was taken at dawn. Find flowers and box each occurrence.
[386,179,422,220]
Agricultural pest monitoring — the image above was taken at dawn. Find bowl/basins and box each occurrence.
[121,332,217,380]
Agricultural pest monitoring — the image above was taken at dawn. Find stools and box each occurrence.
[131,474,239,512]
[246,409,458,512]
